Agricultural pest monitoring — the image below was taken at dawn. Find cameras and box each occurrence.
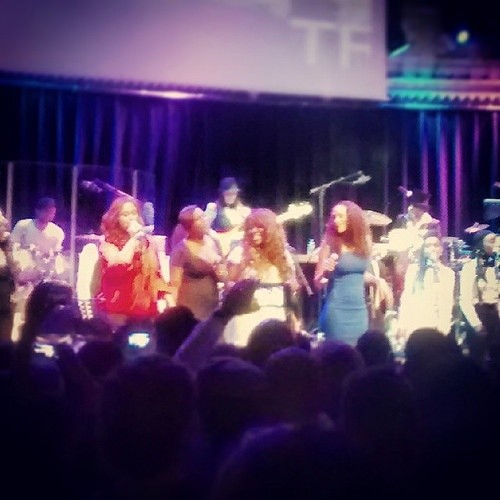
[128,333,150,348]
[253,283,290,307]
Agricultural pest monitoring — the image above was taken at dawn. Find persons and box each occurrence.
[95,196,165,332]
[393,191,442,296]
[169,205,222,323]
[313,200,392,348]
[0,211,21,343]
[9,197,65,342]
[0,279,499,500]
[203,178,253,306]
[394,230,457,364]
[209,207,301,346]
[459,230,500,363]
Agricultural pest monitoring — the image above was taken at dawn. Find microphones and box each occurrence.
[320,253,338,284]
[397,186,413,197]
[81,180,103,193]
[351,175,371,186]
